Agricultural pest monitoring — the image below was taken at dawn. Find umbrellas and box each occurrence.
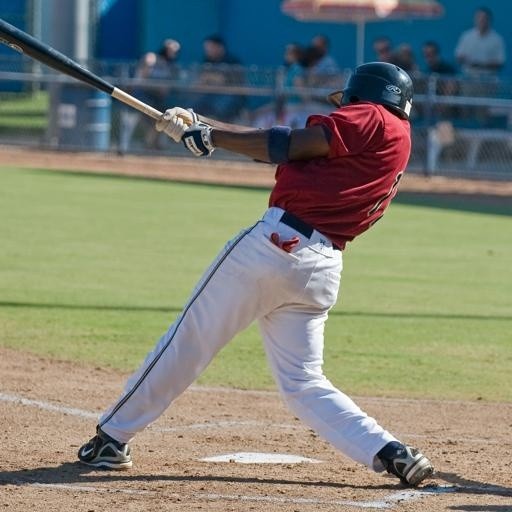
[281,0,443,67]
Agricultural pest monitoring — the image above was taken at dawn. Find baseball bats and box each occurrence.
[0,19,164,120]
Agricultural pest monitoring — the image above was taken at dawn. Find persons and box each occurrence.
[76,60,438,486]
[123,8,504,162]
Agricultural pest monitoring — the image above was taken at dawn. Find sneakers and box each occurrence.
[388,445,433,486]
[78,424,133,469]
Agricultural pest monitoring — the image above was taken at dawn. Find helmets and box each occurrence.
[327,61,415,121]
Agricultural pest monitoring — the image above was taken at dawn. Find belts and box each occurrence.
[280,212,341,250]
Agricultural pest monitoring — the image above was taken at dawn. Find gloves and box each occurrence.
[155,107,199,144]
[182,123,216,157]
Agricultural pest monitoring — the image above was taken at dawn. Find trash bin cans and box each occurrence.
[57,82,111,151]
